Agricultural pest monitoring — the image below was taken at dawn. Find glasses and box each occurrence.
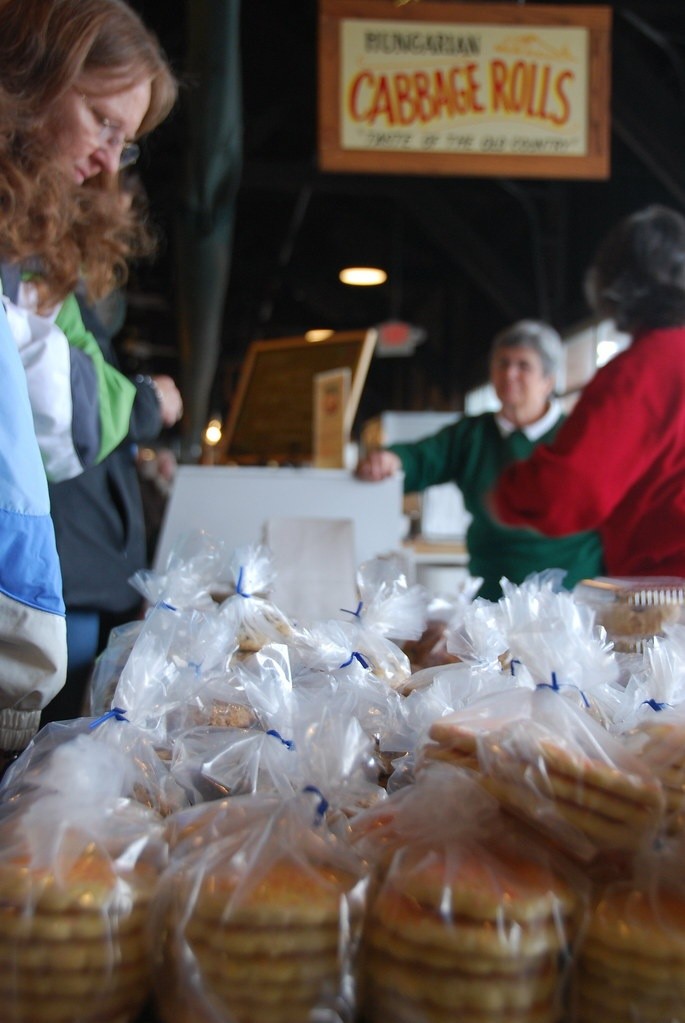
[73,86,141,169]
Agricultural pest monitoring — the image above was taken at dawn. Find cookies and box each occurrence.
[0,599,685,1023]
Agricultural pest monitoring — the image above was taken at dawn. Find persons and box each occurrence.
[485,208,684,585]
[354,323,607,602]
[42,173,182,725]
[0,0,174,779]
